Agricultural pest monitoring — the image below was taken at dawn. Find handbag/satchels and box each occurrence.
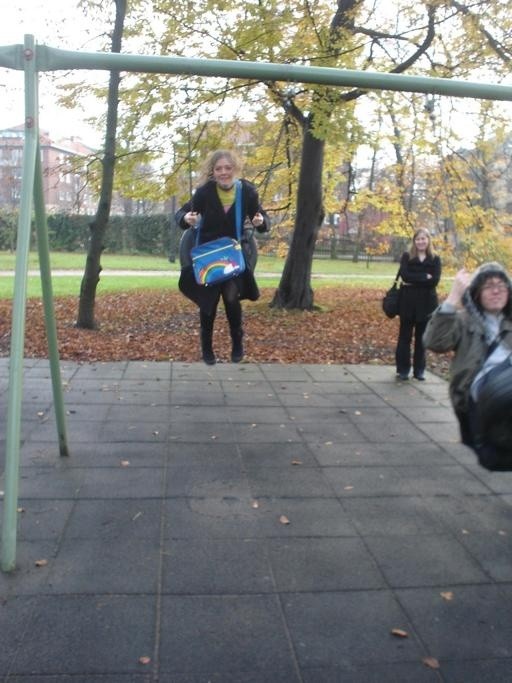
[190,237,245,287]
[383,287,401,318]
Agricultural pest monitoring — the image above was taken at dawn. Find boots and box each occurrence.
[199,313,215,365]
[227,314,243,362]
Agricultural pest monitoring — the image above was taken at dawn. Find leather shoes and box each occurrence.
[400,374,425,380]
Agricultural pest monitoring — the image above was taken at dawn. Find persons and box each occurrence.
[174,149,271,365]
[421,260,512,472]
[395,226,440,380]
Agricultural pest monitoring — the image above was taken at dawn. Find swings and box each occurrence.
[424,93,512,470]
[179,75,299,304]
